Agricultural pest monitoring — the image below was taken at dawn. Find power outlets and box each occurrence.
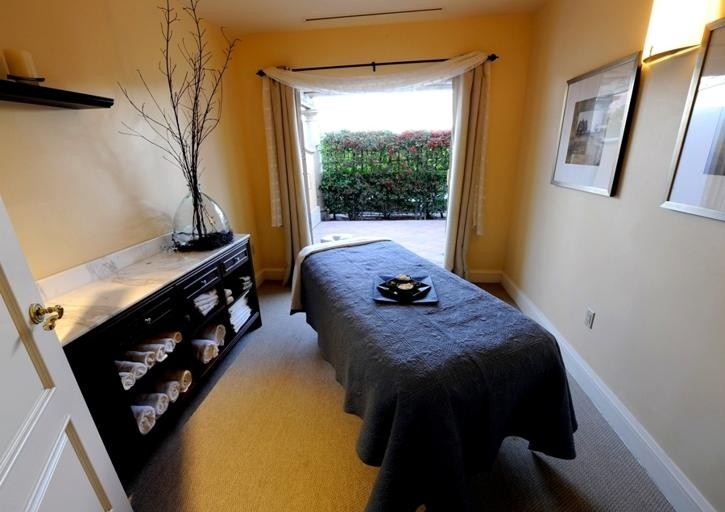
[583,307,596,330]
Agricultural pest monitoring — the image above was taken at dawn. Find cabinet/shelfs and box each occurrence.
[35,223,265,466]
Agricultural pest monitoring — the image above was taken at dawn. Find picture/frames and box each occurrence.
[549,51,643,198]
[658,17,725,224]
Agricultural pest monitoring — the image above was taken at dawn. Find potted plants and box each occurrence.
[113,1,244,253]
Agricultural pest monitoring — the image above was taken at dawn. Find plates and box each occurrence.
[377,274,431,302]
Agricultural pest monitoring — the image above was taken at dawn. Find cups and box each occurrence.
[395,279,416,295]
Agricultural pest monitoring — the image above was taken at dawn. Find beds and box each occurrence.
[290,234,579,512]
